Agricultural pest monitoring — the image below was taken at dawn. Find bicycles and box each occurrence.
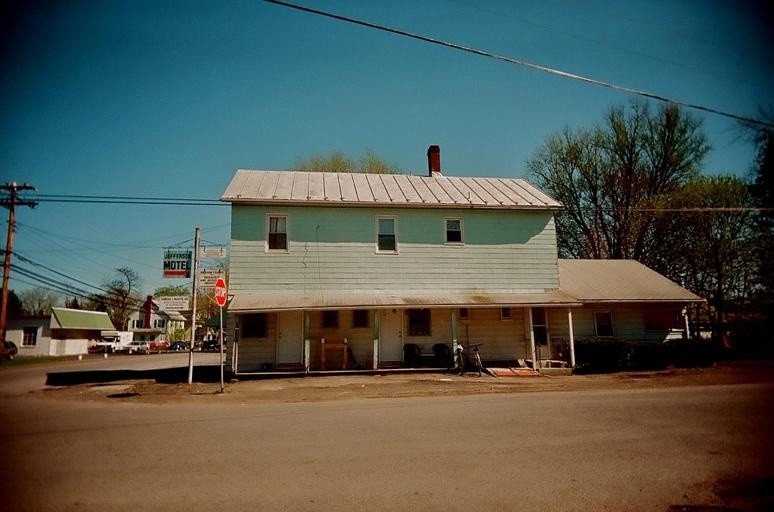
[455,342,470,376]
[468,343,486,377]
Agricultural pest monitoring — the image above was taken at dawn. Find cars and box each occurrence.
[126,340,219,354]
[2,341,18,360]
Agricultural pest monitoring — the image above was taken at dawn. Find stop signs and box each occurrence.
[213,278,228,306]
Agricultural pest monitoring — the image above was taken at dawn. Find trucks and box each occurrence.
[93,331,134,355]
